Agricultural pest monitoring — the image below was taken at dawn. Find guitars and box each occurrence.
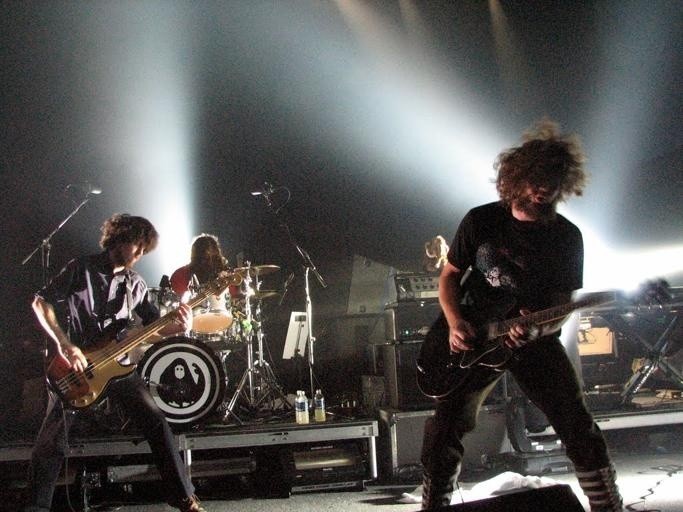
[416,278,666,402]
[46,273,243,408]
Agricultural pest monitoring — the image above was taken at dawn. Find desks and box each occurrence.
[578,300,682,408]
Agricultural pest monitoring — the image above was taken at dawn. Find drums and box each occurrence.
[135,337,227,427]
[190,330,244,350]
[182,281,244,333]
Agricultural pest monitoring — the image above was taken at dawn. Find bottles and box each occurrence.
[293,389,310,426]
[313,389,326,423]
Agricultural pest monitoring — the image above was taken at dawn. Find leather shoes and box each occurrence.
[165,494,207,512]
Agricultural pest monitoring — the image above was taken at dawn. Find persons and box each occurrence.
[26,213,205,512]
[420,120,624,512]
[169,234,237,308]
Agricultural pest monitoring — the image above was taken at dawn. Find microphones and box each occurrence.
[249,186,280,196]
[73,183,102,194]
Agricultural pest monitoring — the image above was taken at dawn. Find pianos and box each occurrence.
[584,287,682,309]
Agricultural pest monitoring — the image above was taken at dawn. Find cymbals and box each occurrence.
[236,291,278,299]
[234,265,280,273]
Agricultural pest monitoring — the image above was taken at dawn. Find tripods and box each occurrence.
[99,199,357,431]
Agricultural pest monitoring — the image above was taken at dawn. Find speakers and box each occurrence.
[376,410,515,483]
[384,302,443,341]
[416,485,587,512]
[380,343,508,411]
